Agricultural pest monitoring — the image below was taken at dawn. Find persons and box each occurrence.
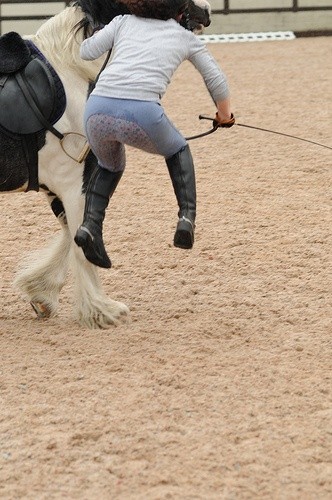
[74,0,231,269]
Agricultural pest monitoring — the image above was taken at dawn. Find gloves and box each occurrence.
[213,112,235,127]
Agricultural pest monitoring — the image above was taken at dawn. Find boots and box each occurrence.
[165,144,197,249]
[74,164,124,268]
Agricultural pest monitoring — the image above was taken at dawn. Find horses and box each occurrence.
[0,0,211,331]
